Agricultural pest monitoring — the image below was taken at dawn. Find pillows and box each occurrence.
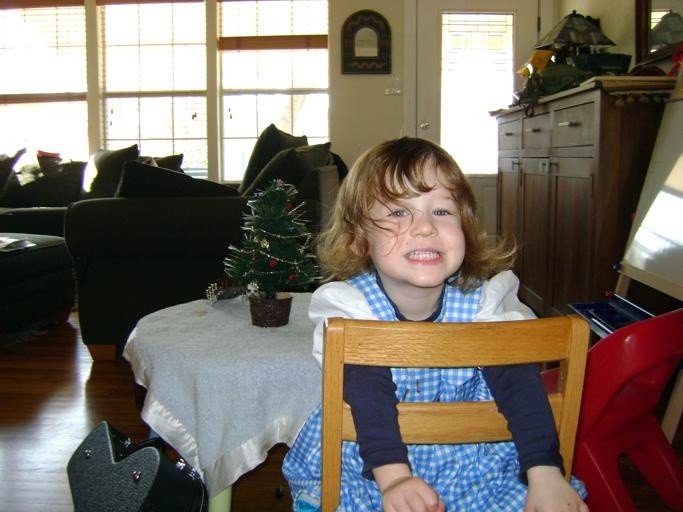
[0,142,183,208]
[239,139,331,196]
[112,157,239,200]
[237,121,309,197]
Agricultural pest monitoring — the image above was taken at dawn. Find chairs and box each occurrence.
[317,316,591,509]
[543,308,682,509]
[64,164,342,365]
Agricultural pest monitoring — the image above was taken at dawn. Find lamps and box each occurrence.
[647,7,682,50]
[530,9,617,67]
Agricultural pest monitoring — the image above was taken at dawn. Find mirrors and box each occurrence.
[339,9,392,75]
[633,0,682,69]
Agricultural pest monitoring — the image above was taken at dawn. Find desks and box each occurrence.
[117,288,320,510]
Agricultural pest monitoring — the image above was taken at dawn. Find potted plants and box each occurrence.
[219,178,320,329]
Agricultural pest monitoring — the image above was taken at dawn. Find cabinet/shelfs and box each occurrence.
[493,75,678,321]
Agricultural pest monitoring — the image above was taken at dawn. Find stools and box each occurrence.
[0,230,75,328]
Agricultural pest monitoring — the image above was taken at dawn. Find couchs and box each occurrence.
[0,169,186,272]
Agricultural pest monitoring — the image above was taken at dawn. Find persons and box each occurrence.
[280,137,592,512]
